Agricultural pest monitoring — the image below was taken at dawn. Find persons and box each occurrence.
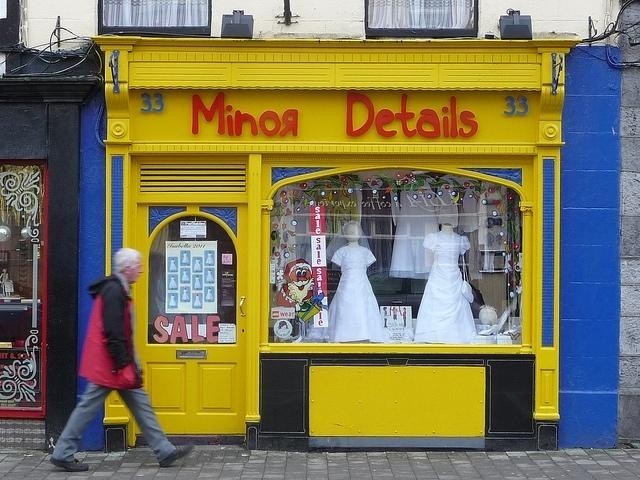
[414,222,477,344]
[49,249,196,472]
[328,220,384,342]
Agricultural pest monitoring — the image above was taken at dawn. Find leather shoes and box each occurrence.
[158,443,193,467]
[50,455,89,472]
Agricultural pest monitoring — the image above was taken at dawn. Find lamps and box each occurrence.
[217,7,255,41]
[495,7,534,43]
